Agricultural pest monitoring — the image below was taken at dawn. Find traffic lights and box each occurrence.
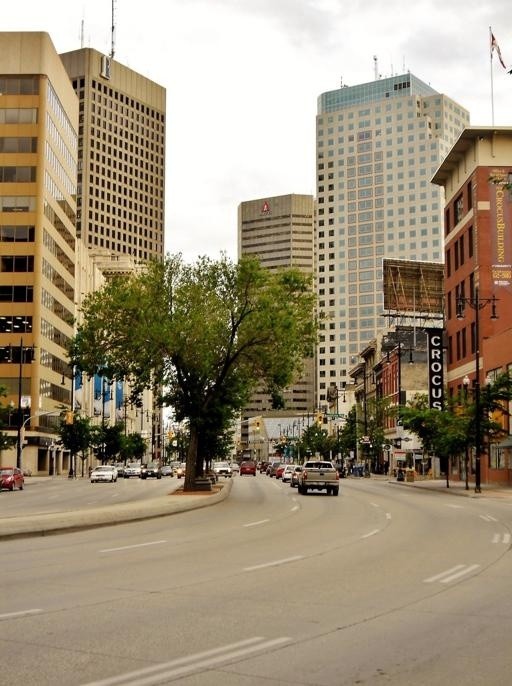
[256,422,261,434]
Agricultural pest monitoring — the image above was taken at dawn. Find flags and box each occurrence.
[490,31,507,67]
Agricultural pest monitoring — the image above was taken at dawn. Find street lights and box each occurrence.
[141,433,167,465]
[457,286,499,492]
[20,408,67,476]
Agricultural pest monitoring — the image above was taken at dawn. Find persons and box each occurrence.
[371,460,389,475]
[89,466,93,478]
[344,467,348,478]
[365,464,369,469]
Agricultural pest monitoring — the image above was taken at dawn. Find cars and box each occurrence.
[213,458,339,496]
[115,461,186,479]
[91,465,119,483]
[0,467,23,492]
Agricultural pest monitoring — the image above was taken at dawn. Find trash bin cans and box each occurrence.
[407,471,415,482]
